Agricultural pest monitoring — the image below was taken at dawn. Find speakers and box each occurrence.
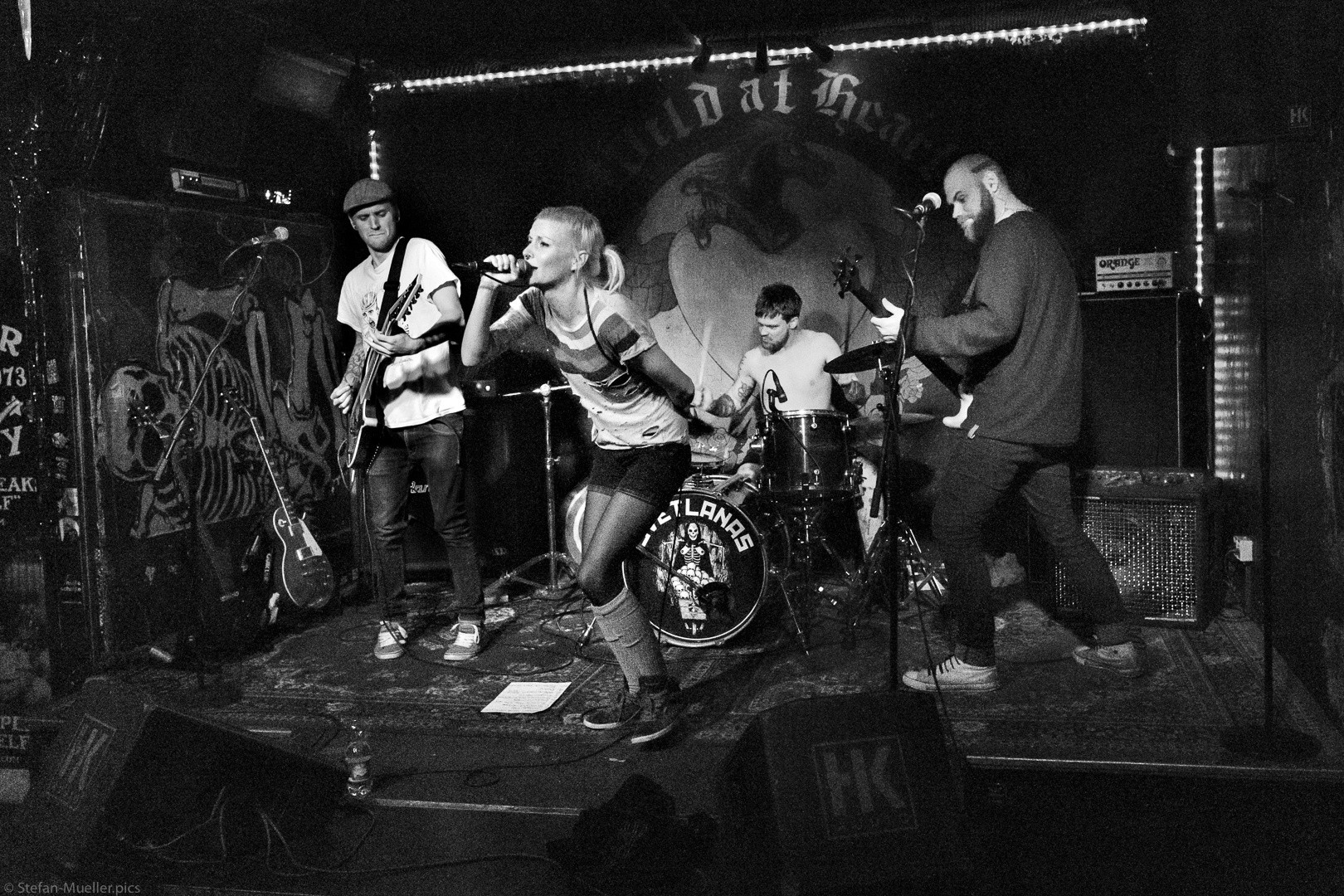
[1071,287,1215,474]
[24,675,348,877]
[700,691,975,896]
[352,398,540,580]
[1026,467,1226,626]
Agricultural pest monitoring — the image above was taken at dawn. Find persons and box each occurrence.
[330,179,486,662]
[680,281,911,611]
[460,206,695,746]
[870,153,1151,691]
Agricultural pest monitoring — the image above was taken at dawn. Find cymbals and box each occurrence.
[822,341,916,375]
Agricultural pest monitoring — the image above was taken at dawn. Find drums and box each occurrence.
[688,450,725,475]
[560,474,589,568]
[762,409,853,499]
[620,472,793,649]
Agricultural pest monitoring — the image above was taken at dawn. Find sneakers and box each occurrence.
[584,674,642,728]
[1071,644,1142,678]
[374,620,409,659]
[444,615,482,660]
[902,656,1001,695]
[631,675,691,743]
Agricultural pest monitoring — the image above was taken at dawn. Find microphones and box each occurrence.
[912,192,941,214]
[465,258,532,278]
[772,372,787,403]
[244,227,289,246]
[709,591,724,608]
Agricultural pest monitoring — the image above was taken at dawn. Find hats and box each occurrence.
[343,178,392,212]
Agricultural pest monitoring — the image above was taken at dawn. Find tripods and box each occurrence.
[477,393,578,603]
[768,351,951,652]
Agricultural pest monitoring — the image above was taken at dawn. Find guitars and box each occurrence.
[219,386,335,612]
[831,247,973,428]
[126,398,261,644]
[345,272,424,471]
